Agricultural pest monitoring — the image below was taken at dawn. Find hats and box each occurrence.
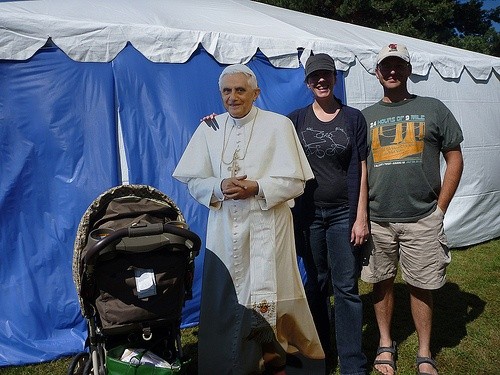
[303,53,336,84]
[375,42,410,69]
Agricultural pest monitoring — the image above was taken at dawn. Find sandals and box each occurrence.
[373,339,397,375]
[415,352,440,375]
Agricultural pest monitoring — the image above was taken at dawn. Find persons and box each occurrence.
[171,63,326,375]
[199,54,369,375]
[361,43,464,375]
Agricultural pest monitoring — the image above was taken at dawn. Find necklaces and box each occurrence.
[221,107,258,178]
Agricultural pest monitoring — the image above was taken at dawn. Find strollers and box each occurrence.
[68,183,202,375]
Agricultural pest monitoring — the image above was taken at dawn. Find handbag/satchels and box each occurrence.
[105,341,182,375]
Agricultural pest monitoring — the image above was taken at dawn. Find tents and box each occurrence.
[0,0,500,367]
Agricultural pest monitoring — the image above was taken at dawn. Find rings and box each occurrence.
[365,239,368,242]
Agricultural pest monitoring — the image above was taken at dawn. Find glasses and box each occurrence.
[376,63,409,71]
